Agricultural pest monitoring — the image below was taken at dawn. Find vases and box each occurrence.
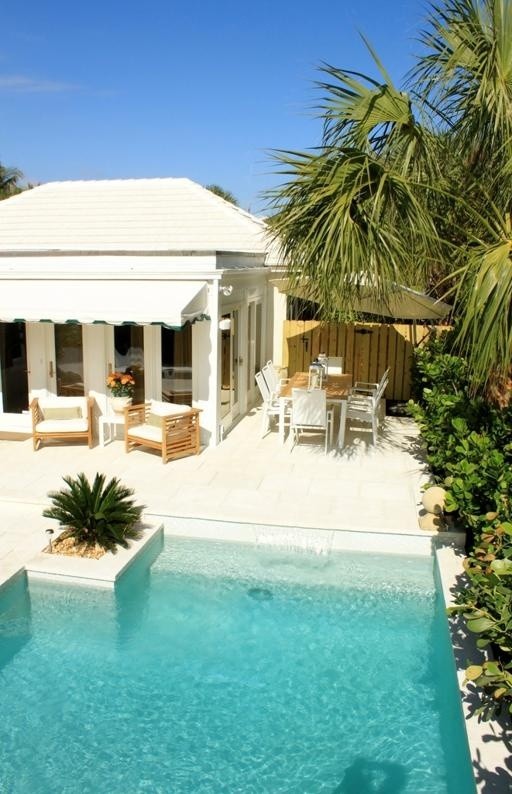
[110,397,133,416]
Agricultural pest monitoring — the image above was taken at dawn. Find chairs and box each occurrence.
[29,396,95,450]
[254,356,391,456]
[124,400,203,464]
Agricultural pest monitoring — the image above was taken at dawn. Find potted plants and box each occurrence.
[25,470,165,590]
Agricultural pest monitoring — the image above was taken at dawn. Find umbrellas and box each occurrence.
[263,268,455,324]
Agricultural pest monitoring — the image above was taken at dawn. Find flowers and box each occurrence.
[105,370,136,397]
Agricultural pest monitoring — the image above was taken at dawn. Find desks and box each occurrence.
[99,411,140,448]
